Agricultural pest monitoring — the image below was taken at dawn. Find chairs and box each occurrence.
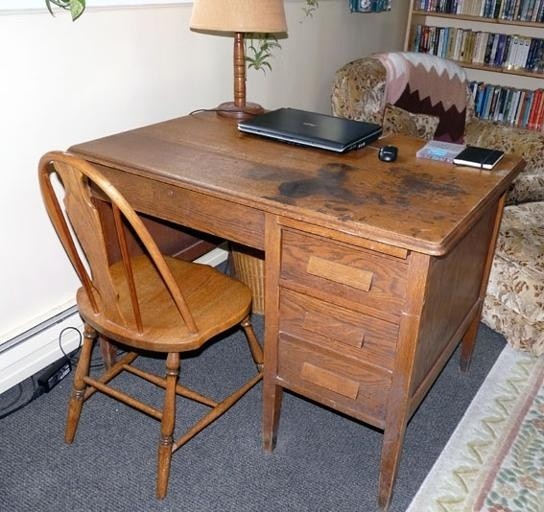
[330,51,544,207]
[38,150,266,500]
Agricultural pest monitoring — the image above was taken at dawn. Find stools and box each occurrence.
[479,200,544,358]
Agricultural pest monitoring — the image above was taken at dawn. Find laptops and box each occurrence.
[237,107,382,153]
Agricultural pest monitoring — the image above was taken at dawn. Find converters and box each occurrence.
[38,358,72,392]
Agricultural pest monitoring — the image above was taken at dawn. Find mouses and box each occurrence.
[378,145,398,162]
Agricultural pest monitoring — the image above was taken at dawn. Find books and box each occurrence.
[452,147,504,171]
[407,1,544,131]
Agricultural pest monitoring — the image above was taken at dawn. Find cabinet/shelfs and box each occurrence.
[403,0,544,78]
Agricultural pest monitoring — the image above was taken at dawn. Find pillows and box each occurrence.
[382,103,441,141]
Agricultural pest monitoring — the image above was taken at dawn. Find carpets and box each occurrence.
[404,341,544,512]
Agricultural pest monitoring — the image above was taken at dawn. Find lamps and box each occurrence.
[188,0,288,121]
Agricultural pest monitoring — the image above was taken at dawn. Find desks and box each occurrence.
[65,106,529,512]
